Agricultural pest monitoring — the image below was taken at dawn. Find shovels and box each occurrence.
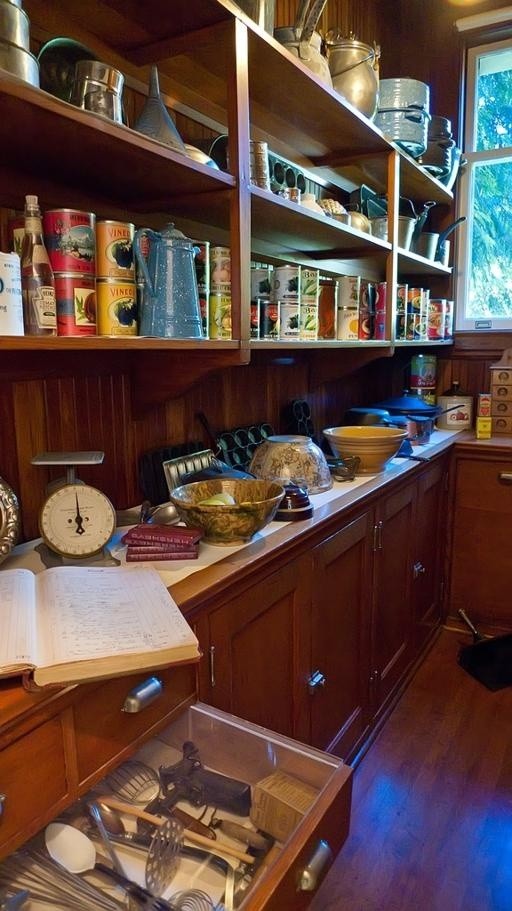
[107,757,216,840]
[45,823,179,911]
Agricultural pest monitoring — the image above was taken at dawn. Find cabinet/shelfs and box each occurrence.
[0,0,455,417]
[453,443,510,644]
[375,455,454,718]
[3,621,207,884]
[207,509,375,762]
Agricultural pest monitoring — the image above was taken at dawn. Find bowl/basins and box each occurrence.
[273,486,314,522]
[323,425,410,475]
[249,434,334,497]
[345,407,390,427]
[169,478,286,549]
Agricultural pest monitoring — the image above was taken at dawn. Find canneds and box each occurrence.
[41,208,233,341]
[409,354,438,406]
[250,264,387,341]
[396,283,455,341]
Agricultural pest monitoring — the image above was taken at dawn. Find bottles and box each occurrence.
[444,381,468,396]
[19,195,59,338]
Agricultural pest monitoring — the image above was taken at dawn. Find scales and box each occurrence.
[30,451,121,569]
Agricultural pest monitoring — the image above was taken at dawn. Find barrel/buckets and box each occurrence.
[368,214,416,250]
[418,231,441,261]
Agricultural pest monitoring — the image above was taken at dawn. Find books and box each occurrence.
[0,564,205,695]
[121,522,207,564]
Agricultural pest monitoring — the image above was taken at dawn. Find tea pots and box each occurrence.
[133,222,206,339]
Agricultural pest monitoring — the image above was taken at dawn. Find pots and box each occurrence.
[374,388,443,431]
[378,77,467,269]
[273,24,379,119]
